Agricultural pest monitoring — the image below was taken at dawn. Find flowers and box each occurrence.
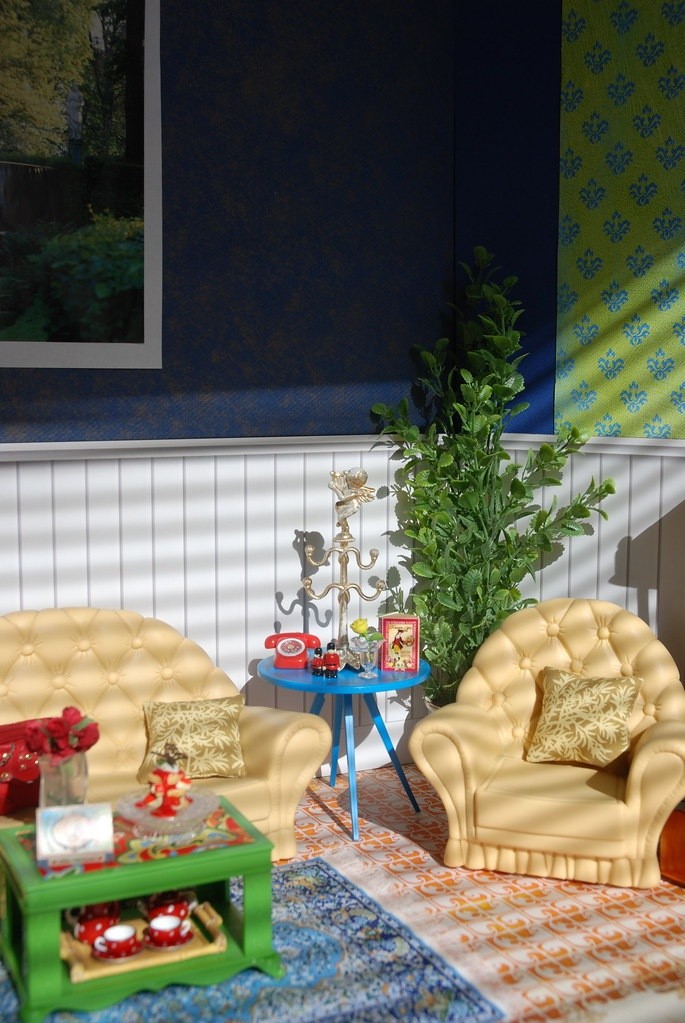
[22,707,101,769]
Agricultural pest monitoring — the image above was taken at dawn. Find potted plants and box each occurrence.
[370,242,617,714]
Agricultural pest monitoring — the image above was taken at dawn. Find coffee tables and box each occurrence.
[0,793,288,1023]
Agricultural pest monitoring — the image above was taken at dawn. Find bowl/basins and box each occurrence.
[117,783,220,847]
[94,925,137,955]
[149,916,191,942]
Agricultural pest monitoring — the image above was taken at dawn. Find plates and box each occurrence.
[92,941,144,962]
[143,930,194,951]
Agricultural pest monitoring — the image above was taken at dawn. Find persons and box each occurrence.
[322,642,340,678]
[310,648,324,676]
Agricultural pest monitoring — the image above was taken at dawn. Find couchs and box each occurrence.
[406,598,685,891]
[0,606,332,863]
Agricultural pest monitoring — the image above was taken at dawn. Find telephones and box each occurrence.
[265,633,321,669]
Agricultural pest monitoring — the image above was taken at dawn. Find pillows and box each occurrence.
[137,694,249,784]
[526,666,645,778]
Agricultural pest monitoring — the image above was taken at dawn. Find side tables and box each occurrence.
[256,648,430,841]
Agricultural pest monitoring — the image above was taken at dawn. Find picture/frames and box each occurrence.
[0,0,164,370]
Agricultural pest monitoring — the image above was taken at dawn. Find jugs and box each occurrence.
[66,898,120,946]
[136,889,198,921]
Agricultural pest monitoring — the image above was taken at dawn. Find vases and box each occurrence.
[33,750,91,809]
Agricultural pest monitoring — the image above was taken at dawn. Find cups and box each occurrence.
[38,750,88,805]
[358,652,378,679]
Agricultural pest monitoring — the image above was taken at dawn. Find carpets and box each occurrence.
[0,841,525,1023]
